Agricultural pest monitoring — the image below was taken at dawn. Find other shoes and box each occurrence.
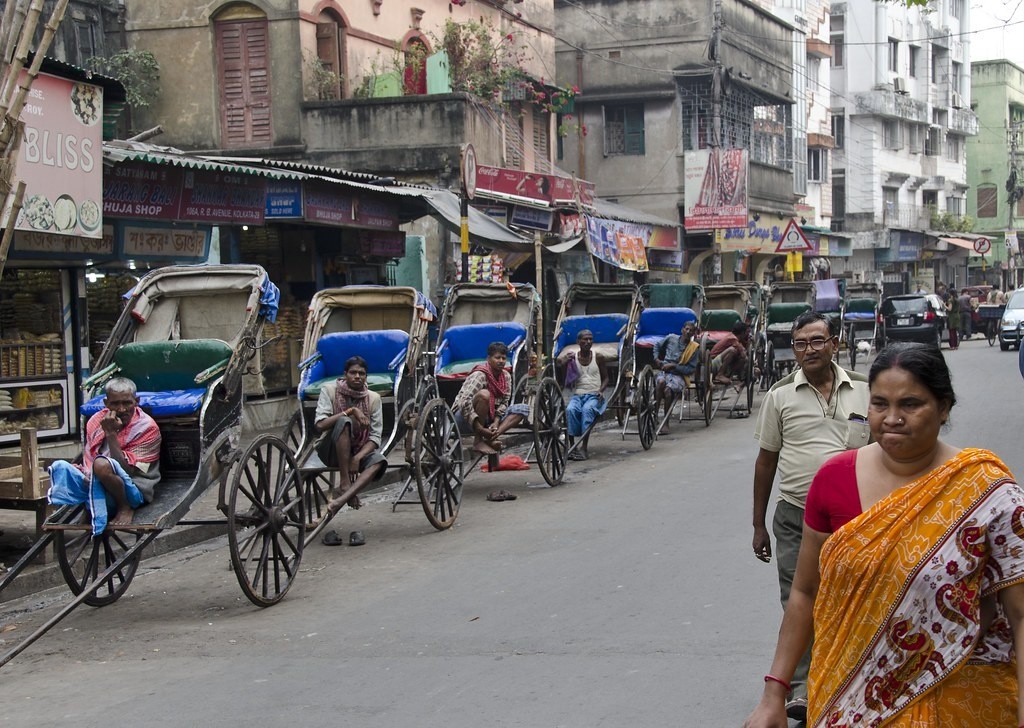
[728,411,749,418]
[659,424,670,435]
[569,447,589,461]
[487,489,518,501]
[349,531,365,546]
[323,530,342,545]
[785,699,807,721]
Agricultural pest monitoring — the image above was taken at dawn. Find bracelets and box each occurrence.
[764,675,792,695]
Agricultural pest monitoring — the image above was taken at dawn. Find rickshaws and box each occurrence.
[841,283,886,370]
[516,283,644,474]
[614,284,712,445]
[390,283,570,513]
[809,278,848,366]
[675,279,767,420]
[0,265,310,670]
[227,280,466,571]
[755,279,819,392]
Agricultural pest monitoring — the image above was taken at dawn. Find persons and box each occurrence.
[752,310,870,611]
[913,283,927,295]
[651,321,701,434]
[515,174,552,201]
[711,322,747,385]
[451,342,531,455]
[986,284,1007,305]
[713,149,747,215]
[739,343,1023,728]
[1005,284,1016,302]
[958,289,975,340]
[559,329,609,461]
[49,376,162,525]
[314,356,388,518]
[943,288,960,350]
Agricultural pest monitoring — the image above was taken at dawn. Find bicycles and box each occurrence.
[986,316,1000,347]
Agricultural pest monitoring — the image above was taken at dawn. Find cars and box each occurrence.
[959,285,993,339]
[874,293,951,352]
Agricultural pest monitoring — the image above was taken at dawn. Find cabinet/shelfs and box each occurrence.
[0,267,70,444]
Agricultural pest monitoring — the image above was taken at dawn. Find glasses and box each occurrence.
[790,335,834,351]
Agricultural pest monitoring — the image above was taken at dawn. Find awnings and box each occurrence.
[921,237,974,251]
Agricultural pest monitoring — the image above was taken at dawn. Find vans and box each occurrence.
[998,289,1024,351]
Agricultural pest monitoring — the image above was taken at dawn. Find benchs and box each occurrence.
[695,310,743,344]
[304,329,413,401]
[80,337,234,427]
[768,302,814,332]
[814,296,843,319]
[844,298,878,320]
[635,306,698,349]
[554,312,631,366]
[436,322,528,384]
